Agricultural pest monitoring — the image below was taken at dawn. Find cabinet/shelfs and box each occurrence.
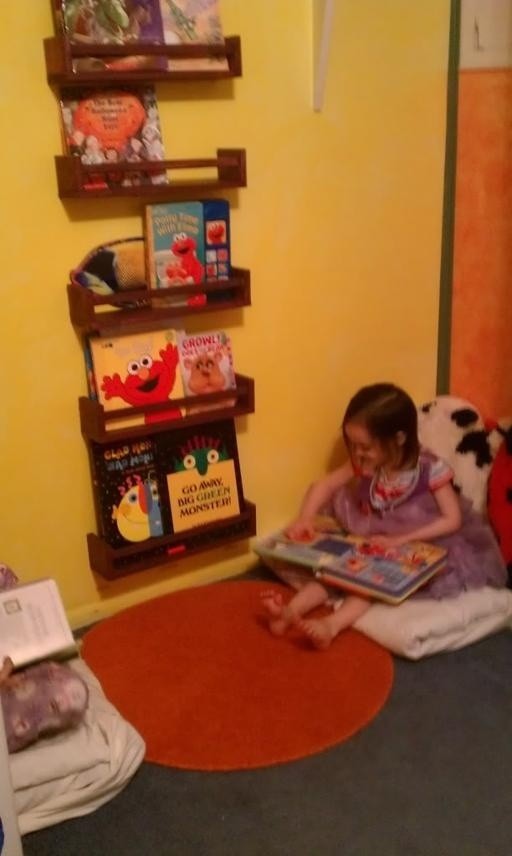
[44,35,257,579]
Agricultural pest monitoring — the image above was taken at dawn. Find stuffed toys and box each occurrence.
[415,393,511,512]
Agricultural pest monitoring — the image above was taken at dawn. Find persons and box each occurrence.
[257,382,509,650]
[0,558,87,753]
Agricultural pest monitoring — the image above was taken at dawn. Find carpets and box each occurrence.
[75,577,395,771]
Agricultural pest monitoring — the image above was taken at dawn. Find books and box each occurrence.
[257,518,449,606]
[0,576,79,674]
